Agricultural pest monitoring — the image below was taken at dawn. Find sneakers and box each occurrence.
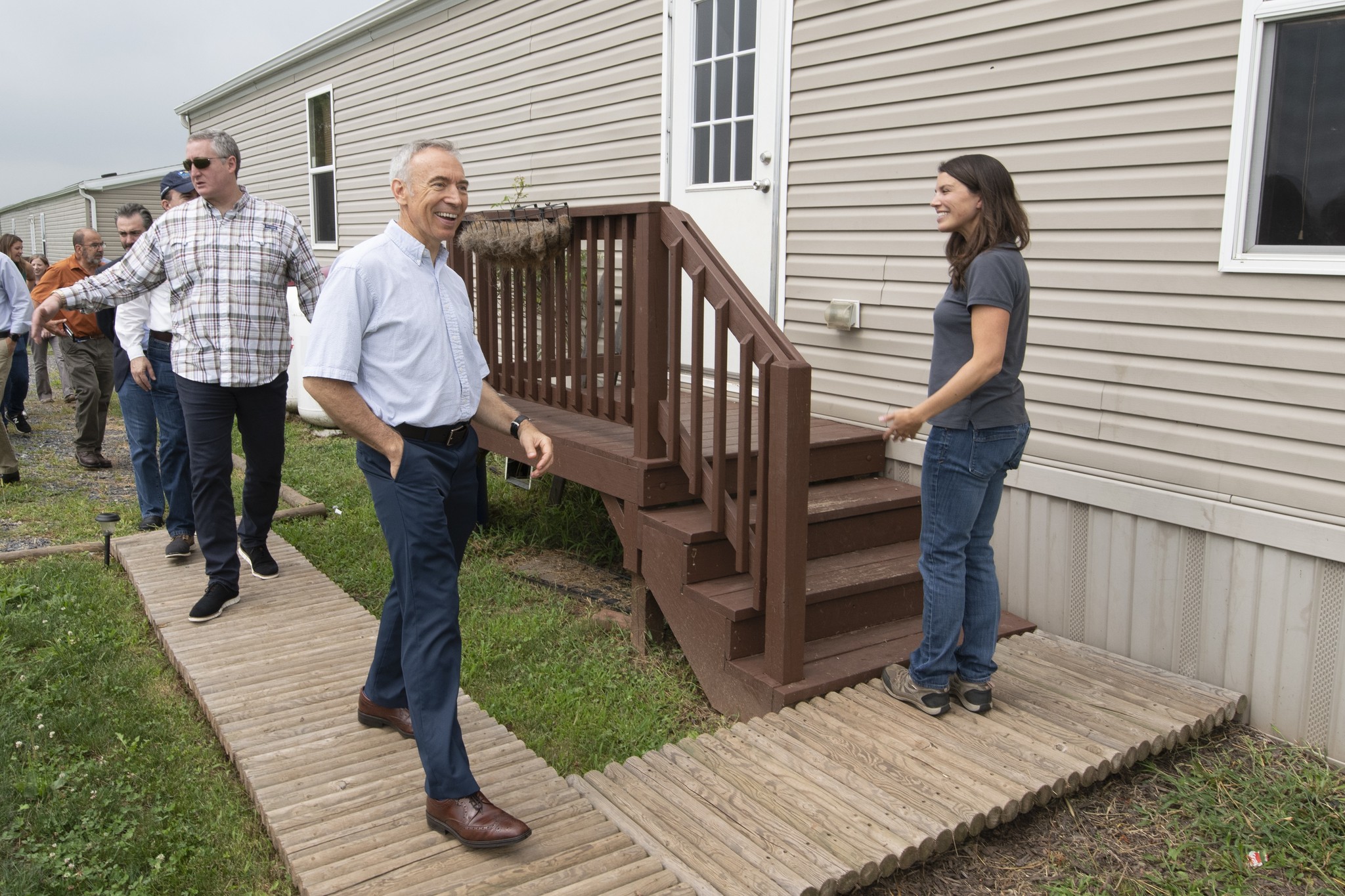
[238,542,279,579]
[948,672,995,713]
[188,581,241,621]
[165,534,196,558]
[64,394,76,403]
[882,662,952,716]
[41,399,54,404]
[5,411,31,433]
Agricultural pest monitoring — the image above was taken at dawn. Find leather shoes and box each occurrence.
[137,515,164,531]
[75,450,112,469]
[0,470,20,483]
[358,686,415,739]
[425,790,532,848]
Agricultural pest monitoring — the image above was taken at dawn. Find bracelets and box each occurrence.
[510,415,532,440]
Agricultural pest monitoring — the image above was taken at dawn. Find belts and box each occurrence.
[391,421,471,446]
[149,329,173,342]
[75,334,105,341]
[0,331,10,338]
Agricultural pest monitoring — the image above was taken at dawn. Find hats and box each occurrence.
[160,170,195,199]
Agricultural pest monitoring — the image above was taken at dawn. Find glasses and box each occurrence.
[80,243,106,249]
[183,157,226,171]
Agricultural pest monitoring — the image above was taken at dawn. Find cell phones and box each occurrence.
[62,322,75,342]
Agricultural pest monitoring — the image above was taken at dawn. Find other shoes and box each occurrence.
[22,410,27,419]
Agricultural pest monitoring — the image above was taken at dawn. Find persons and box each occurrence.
[880,154,1031,715]
[304,140,555,846]
[0,169,201,558]
[32,129,325,620]
